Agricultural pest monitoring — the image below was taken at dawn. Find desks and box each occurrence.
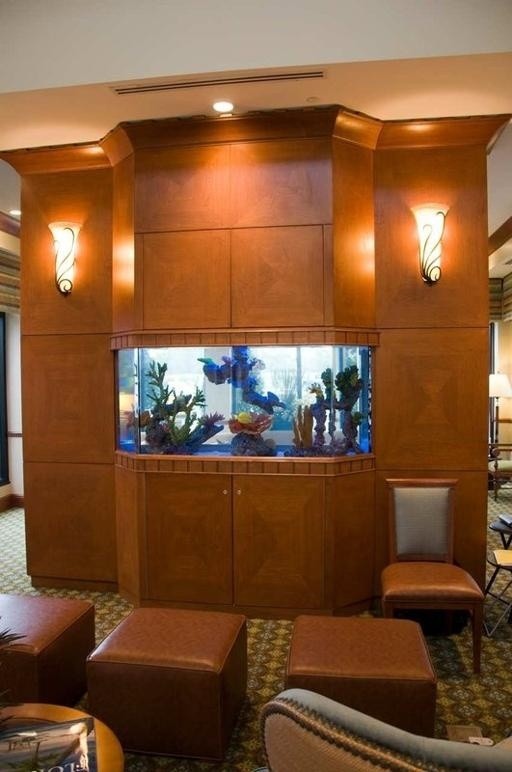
[1,696,129,772]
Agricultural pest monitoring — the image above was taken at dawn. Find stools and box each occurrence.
[2,588,100,714]
[85,603,250,762]
[280,607,440,738]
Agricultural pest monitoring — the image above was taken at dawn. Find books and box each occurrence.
[500,563,512,568]
[491,548,512,566]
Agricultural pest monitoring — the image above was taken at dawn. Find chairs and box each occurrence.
[483,546,511,637]
[489,518,511,550]
[485,441,512,502]
[253,682,511,772]
[372,471,490,680]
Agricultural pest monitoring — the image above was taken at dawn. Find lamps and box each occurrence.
[45,219,84,294]
[488,367,512,449]
[410,199,452,286]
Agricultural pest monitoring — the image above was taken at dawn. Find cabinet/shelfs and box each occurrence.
[110,463,378,619]
